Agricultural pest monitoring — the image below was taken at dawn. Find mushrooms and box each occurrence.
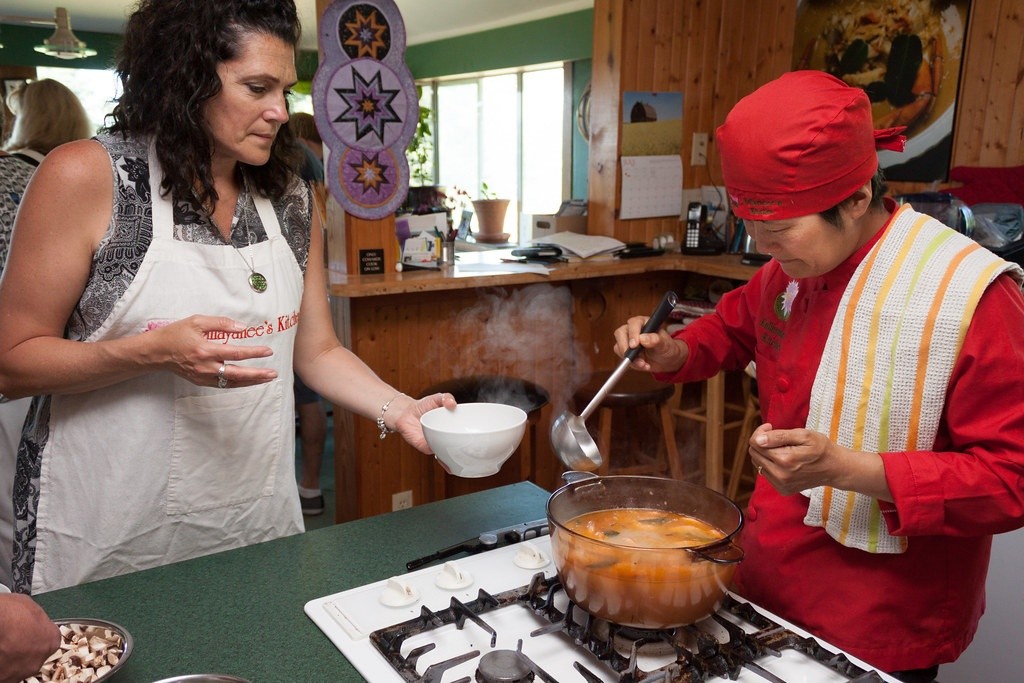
[19,625,124,683]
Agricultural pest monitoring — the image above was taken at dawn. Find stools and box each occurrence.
[653,325,762,503]
[590,365,688,482]
[418,375,551,505]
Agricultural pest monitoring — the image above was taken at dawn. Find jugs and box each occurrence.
[894,192,976,241]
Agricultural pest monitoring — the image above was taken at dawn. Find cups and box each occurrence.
[438,242,454,265]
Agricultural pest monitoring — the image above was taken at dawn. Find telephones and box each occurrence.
[682,202,723,255]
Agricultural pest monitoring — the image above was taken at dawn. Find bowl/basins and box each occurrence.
[17,617,134,683]
[152,673,252,683]
[419,402,528,479]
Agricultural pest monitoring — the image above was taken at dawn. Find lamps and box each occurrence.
[33,6,97,60]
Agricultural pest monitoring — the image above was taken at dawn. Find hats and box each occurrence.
[716,69,909,220]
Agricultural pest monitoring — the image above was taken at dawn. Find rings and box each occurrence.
[218,364,227,380]
[758,467,763,476]
[218,378,227,389]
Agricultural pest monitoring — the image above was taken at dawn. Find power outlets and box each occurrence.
[392,490,413,512]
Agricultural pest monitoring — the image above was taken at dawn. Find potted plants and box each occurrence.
[470,182,510,245]
[403,85,448,209]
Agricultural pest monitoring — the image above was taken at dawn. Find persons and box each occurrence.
[0,592,62,683]
[0,1,457,598]
[0,80,90,583]
[274,109,331,515]
[616,70,1024,683]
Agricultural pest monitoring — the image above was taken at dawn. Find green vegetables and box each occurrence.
[832,37,869,80]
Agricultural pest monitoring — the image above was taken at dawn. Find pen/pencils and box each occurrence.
[500,259,527,263]
[556,256,568,263]
[434,226,458,241]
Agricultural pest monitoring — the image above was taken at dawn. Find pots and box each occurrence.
[545,470,746,630]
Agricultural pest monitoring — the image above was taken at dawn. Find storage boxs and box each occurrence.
[531,213,588,240]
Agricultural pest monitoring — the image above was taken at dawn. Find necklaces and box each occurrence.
[189,169,267,293]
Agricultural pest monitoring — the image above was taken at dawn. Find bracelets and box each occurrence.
[378,392,403,439]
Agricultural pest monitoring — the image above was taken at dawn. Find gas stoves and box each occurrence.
[303,531,906,682]
